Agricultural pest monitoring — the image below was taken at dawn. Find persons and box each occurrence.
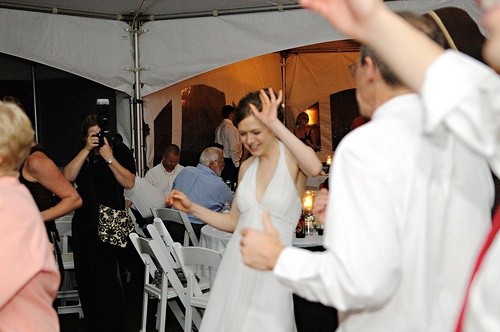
[288,111,316,152]
[165,146,235,247]
[239,9,500,332]
[161,86,323,332]
[0,101,60,332]
[62,111,137,332]
[19,96,82,314]
[125,143,185,238]
[298,0,500,332]
[215,105,243,192]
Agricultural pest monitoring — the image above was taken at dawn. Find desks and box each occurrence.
[194,226,323,280]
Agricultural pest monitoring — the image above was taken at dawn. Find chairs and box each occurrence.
[173,242,222,332]
[53,221,84,319]
[146,207,214,314]
[128,232,201,332]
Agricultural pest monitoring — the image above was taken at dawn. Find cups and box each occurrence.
[317,222,324,236]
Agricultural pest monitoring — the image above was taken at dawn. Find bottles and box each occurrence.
[222,202,230,214]
[296,210,305,239]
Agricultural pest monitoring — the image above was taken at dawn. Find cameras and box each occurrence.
[90,99,123,151]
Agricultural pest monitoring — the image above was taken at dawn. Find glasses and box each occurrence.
[302,117,309,120]
[347,59,367,79]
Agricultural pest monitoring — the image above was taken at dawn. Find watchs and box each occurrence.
[105,156,115,166]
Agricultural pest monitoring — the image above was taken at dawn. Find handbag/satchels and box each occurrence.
[99,205,136,248]
